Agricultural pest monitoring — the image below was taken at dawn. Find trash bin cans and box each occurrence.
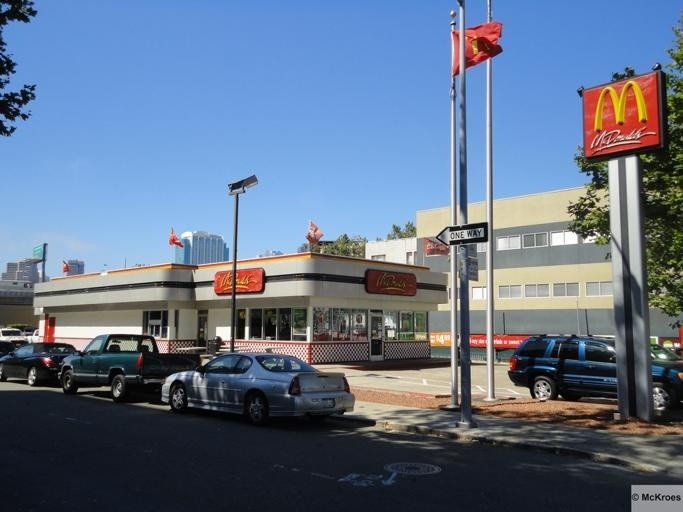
[207,336,222,356]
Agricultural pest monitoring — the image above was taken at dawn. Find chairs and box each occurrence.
[109,345,149,351]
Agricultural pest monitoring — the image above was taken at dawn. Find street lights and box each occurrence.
[226,174,259,352]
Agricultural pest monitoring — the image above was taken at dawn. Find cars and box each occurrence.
[0,327,355,427]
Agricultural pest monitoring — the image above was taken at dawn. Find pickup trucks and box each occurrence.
[508,334,683,417]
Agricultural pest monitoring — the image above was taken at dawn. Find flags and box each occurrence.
[449,22,504,79]
